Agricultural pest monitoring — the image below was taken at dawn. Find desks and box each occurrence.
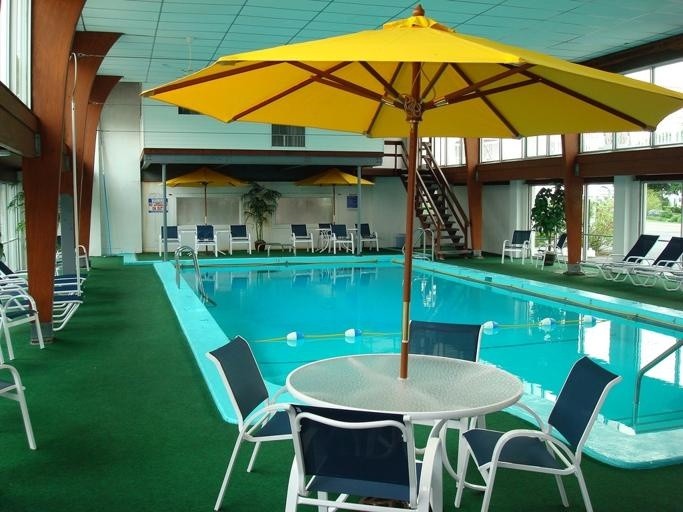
[284,353,525,500]
[316,227,358,254]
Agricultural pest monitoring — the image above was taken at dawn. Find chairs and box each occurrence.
[160,225,181,259]
[0,248,88,364]
[204,333,305,511]
[279,411,443,512]
[405,320,488,490]
[454,355,623,512]
[0,354,37,450]
[580,234,659,284]
[501,229,533,265]
[331,225,354,254]
[619,237,683,285]
[195,224,218,257]
[229,223,252,255]
[658,261,683,293]
[317,223,336,252]
[535,233,570,271]
[355,223,380,255]
[290,223,315,255]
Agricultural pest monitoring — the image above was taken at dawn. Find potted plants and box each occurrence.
[531,186,571,266]
[242,184,281,251]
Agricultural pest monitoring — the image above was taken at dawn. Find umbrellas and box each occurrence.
[159,166,250,256]
[139,5,682,512]
[296,169,376,251]
